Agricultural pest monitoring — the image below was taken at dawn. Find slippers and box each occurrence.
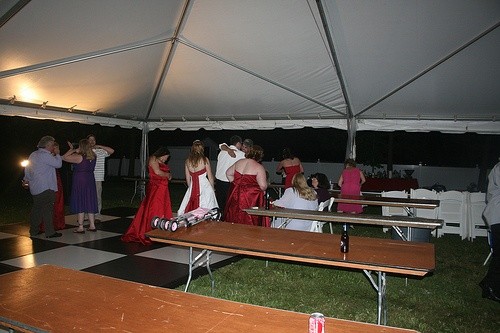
[73,230,85,233]
[87,227,96,231]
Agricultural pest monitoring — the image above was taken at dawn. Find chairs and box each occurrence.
[381,190,408,234]
[411,187,490,242]
[310,198,338,233]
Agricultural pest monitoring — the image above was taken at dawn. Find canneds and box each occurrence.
[308,313,325,333]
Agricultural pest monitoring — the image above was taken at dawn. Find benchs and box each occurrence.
[242,206,444,241]
[319,194,441,220]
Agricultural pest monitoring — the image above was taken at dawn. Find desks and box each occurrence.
[0,264,418,333]
[123,177,191,207]
[145,220,435,325]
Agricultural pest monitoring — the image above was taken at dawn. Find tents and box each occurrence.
[1,0,499,162]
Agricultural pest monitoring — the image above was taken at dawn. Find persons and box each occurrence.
[311,172,330,224]
[29,142,66,233]
[338,158,366,232]
[214,134,271,229]
[76,134,115,226]
[478,160,500,302]
[176,138,220,218]
[62,139,99,233]
[120,147,174,246]
[21,136,62,238]
[276,147,304,197]
[269,173,323,234]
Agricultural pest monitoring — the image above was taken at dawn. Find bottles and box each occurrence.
[340,224,349,253]
[265,193,270,210]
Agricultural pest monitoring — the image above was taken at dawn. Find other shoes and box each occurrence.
[47,233,62,238]
[94,219,99,223]
[480,282,487,289]
